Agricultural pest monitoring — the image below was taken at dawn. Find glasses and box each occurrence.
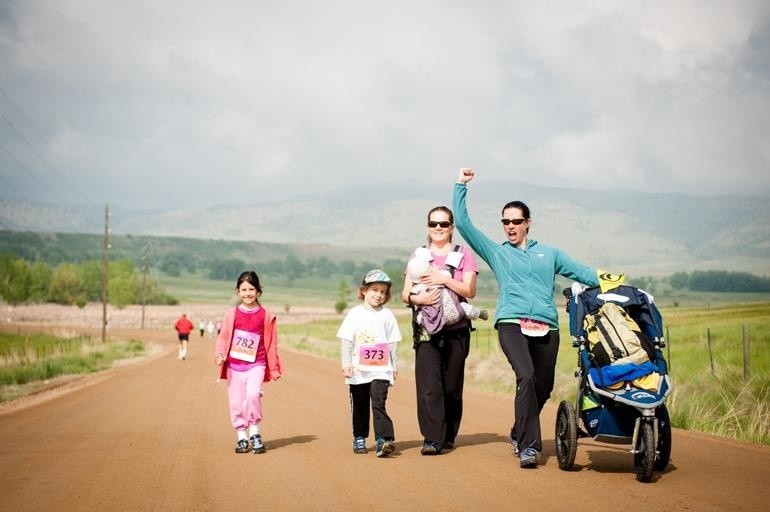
[428,221,451,228]
[501,219,526,225]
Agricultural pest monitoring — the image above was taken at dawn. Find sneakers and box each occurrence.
[236,439,248,453]
[376,439,395,458]
[508,433,521,457]
[250,434,265,454]
[520,448,538,468]
[421,441,443,454]
[442,442,453,449]
[353,437,367,454]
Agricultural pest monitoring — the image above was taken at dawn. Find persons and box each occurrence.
[214,271,283,454]
[335,269,401,458]
[175,313,223,361]
[401,206,479,454]
[451,166,600,469]
[406,256,488,342]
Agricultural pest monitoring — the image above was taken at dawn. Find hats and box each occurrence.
[407,256,431,284]
[362,270,392,286]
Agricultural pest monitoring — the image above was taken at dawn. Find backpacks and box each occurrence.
[583,302,642,368]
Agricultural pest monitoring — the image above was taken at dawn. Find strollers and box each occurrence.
[553,281,675,484]
